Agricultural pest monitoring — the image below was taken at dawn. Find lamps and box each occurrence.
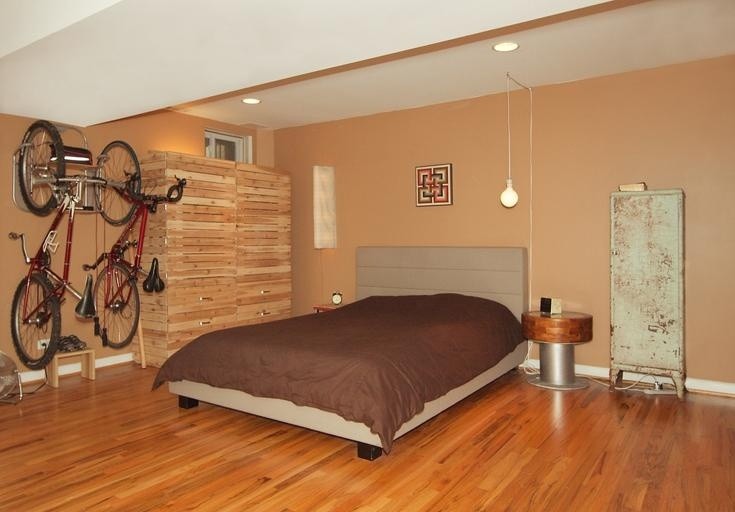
[500,71,519,210]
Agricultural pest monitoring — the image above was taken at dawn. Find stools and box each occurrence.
[46,349,96,389]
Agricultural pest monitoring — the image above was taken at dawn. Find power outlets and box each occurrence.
[38,339,50,350]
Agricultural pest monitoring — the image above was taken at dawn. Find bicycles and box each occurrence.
[8,119,146,371]
[83,140,187,351]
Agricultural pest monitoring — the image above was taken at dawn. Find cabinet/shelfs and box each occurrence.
[610,190,687,400]
[131,150,292,369]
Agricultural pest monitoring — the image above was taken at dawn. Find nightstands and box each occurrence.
[310,304,343,313]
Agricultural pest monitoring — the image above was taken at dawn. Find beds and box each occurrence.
[169,246,529,462]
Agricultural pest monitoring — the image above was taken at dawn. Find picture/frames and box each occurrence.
[412,164,453,207]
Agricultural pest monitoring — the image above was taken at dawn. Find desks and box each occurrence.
[522,311,596,392]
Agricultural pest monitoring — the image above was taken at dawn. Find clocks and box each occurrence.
[332,290,343,305]
